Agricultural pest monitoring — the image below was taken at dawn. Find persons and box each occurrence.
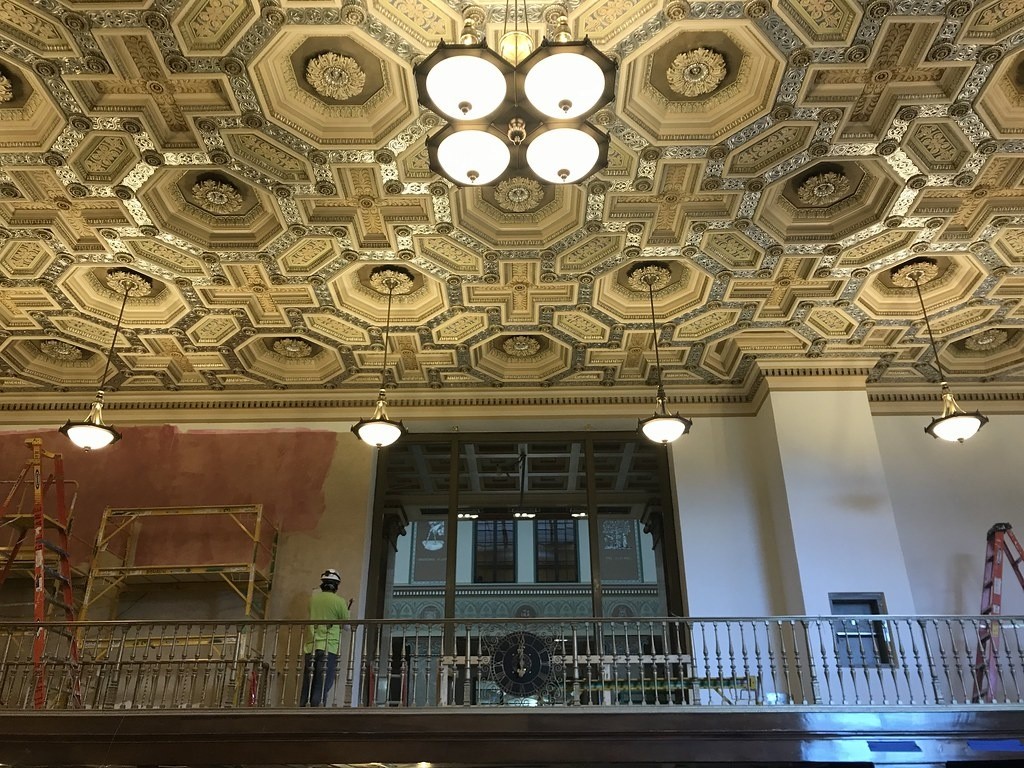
[300,568,349,708]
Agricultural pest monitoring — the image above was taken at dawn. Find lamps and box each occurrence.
[891,257,990,444]
[628,262,694,446]
[350,264,416,450]
[59,268,153,452]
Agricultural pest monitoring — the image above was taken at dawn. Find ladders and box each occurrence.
[1,437,82,710]
[973,521,1023,704]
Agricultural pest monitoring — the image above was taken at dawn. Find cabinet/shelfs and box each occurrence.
[50,504,279,705]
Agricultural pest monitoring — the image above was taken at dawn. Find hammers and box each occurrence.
[348,598,354,609]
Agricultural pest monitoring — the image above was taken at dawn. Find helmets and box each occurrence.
[320,569,341,582]
